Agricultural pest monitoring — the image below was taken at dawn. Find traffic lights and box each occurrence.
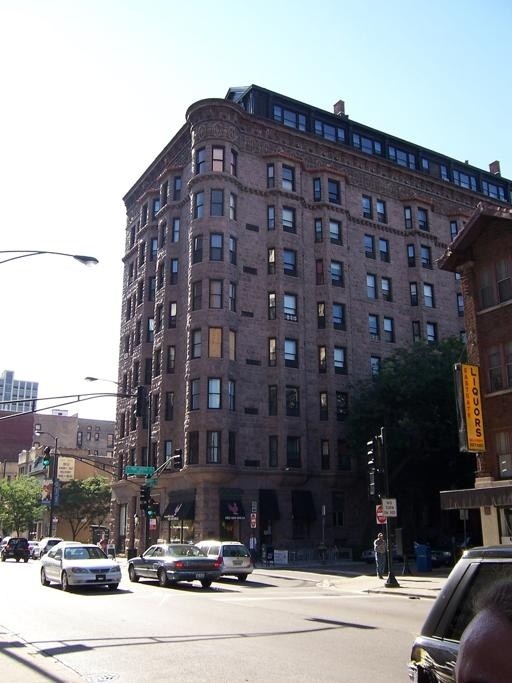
[147,503,158,518]
[43,446,51,465]
[174,449,183,468]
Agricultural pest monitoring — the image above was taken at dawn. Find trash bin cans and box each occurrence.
[127,548,137,560]
[415,545,432,573]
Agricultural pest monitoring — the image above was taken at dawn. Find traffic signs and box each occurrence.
[251,500,257,512]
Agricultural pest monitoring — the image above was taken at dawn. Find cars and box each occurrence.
[28,540,39,556]
[0,535,30,562]
[407,544,511,683]
[362,538,431,565]
[430,546,472,567]
[32,537,64,559]
[194,539,254,582]
[128,544,223,587]
[40,542,122,591]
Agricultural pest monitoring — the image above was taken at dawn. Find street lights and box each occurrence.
[86,376,153,550]
[35,429,58,536]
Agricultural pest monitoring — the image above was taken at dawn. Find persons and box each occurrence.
[454,578,512,682]
[28,533,44,541]
[373,533,387,579]
[249,534,257,562]
[97,538,108,555]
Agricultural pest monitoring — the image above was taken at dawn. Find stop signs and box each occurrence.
[375,504,388,524]
[251,513,257,528]
